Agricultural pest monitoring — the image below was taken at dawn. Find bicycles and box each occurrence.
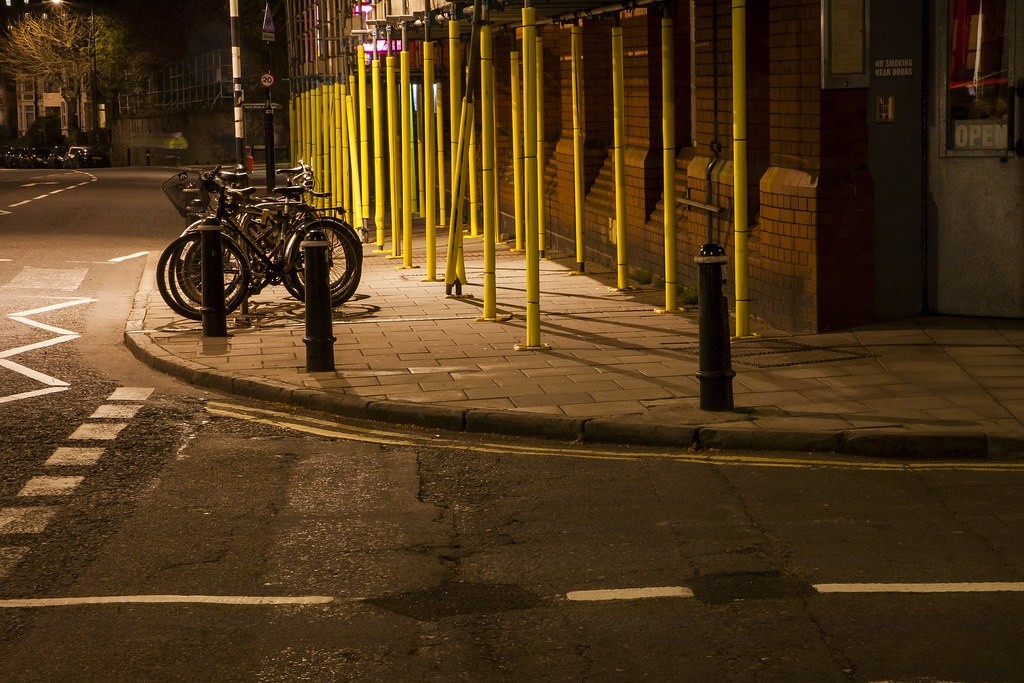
[156,159,363,321]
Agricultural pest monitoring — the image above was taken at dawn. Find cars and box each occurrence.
[47,146,68,168]
[25,147,50,168]
[3,148,24,167]
[71,148,109,168]
[19,149,29,167]
[62,146,84,168]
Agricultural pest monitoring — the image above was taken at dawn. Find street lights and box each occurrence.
[51,0,98,146]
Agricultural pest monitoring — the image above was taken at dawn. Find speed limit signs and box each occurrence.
[260,72,275,87]
